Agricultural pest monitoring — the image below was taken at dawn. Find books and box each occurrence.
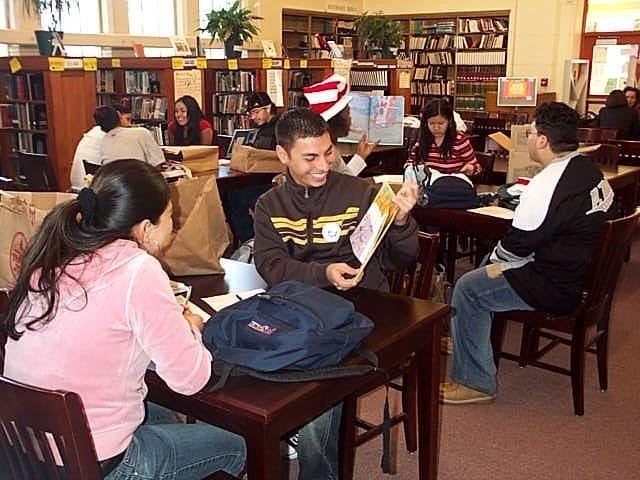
[410,35,456,49]
[459,19,509,33]
[292,31,353,107]
[1,69,289,187]
[409,19,456,33]
[351,70,390,86]
[336,95,405,145]
[458,34,507,49]
[456,81,498,110]
[457,51,506,64]
[349,181,400,281]
[413,51,455,64]
[457,65,506,80]
[353,58,412,68]
[411,66,455,108]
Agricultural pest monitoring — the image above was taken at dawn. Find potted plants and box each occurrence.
[194,1,266,58]
[25,1,70,56]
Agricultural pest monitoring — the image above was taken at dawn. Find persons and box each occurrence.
[444,96,467,132]
[404,99,482,273]
[435,103,618,407]
[253,105,421,298]
[305,74,381,177]
[1,158,250,462]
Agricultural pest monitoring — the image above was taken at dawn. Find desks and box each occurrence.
[486,91,556,125]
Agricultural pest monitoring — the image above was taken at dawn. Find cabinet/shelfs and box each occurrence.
[0,56,413,194]
[282,8,510,128]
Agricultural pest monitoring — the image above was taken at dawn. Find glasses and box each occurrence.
[526,128,541,138]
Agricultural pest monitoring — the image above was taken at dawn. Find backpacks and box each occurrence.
[202,279,380,395]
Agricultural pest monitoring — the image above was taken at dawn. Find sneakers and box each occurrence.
[439,381,497,405]
[441,336,453,355]
[280,431,299,460]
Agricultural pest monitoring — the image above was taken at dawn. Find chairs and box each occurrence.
[0,120,640,480]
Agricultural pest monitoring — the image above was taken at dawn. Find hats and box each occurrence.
[304,72,353,122]
[245,92,271,113]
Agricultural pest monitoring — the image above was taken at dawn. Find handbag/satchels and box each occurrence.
[497,176,534,211]
[403,161,481,210]
